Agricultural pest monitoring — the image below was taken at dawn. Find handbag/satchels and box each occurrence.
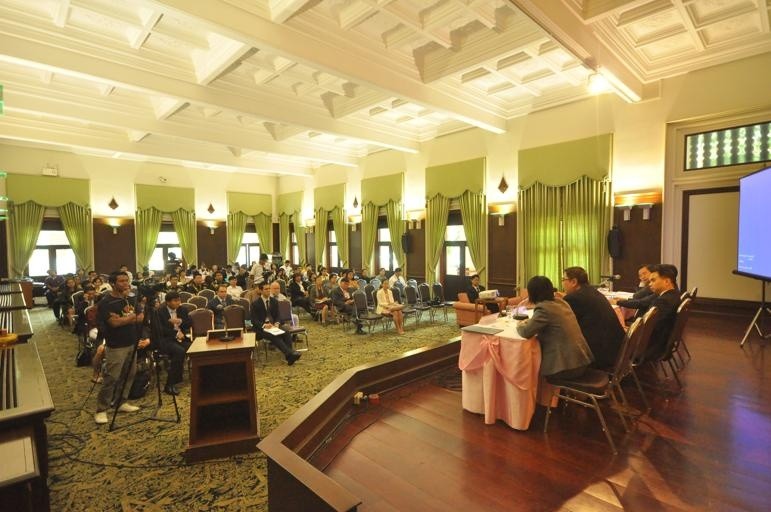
[130,372,149,399]
[386,302,405,311]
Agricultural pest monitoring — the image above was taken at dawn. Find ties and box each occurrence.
[266,300,269,311]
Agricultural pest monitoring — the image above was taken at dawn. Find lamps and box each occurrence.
[491,212,511,226]
[638,202,655,222]
[305,217,316,232]
[403,210,424,231]
[349,214,362,232]
[208,224,219,235]
[111,224,121,235]
[616,203,635,221]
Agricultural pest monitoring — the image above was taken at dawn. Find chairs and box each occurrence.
[178,303,197,339]
[433,282,454,323]
[456,292,508,328]
[307,284,329,323]
[370,288,398,329]
[275,299,309,349]
[542,287,700,456]
[389,287,417,327]
[419,282,445,323]
[84,305,111,372]
[46,260,419,301]
[330,288,351,332]
[187,295,208,309]
[403,285,432,325]
[188,308,215,372]
[353,289,386,337]
[231,297,250,316]
[509,290,529,308]
[222,305,258,359]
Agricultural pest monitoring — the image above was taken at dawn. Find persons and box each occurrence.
[288,265,406,335]
[93,272,144,425]
[44,251,301,394]
[469,274,499,313]
[516,263,681,387]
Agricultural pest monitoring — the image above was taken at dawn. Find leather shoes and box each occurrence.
[118,403,138,412]
[287,350,300,364]
[165,384,179,395]
[95,411,108,424]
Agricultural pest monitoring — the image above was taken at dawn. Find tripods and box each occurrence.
[108,300,180,431]
[740,280,771,347]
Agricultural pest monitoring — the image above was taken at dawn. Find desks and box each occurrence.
[459,289,639,431]
[474,296,506,323]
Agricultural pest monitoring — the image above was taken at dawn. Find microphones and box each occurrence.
[217,304,235,341]
[513,288,557,320]
[113,287,123,293]
[600,274,622,279]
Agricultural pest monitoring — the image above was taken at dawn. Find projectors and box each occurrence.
[479,289,498,299]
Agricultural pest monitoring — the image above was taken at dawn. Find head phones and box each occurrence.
[110,270,130,286]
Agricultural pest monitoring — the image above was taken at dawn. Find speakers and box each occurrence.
[402,234,411,254]
[608,226,623,257]
